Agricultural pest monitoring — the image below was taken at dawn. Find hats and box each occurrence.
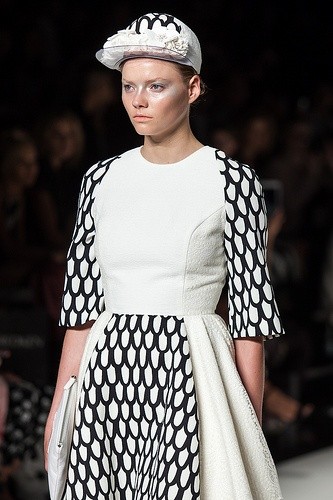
[98,13,204,71]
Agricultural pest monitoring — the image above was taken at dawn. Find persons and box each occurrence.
[43,13,285,499]
[0,54,333,498]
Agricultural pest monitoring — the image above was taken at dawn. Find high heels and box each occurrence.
[261,388,322,430]
